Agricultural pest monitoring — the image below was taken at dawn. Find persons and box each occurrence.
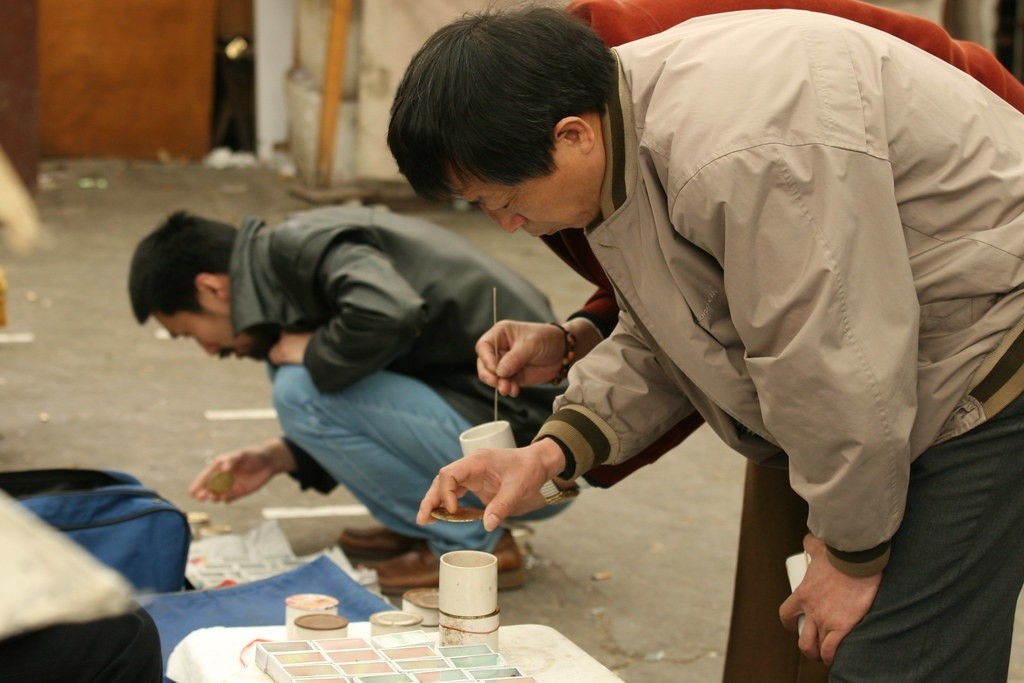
[128,206,579,594]
[387,9,1023,682]
[475,1,1023,400]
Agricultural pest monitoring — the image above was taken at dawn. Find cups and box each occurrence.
[402,587,439,632]
[460,421,517,457]
[439,552,500,655]
[369,611,424,638]
[292,614,348,640]
[786,552,811,640]
[285,593,339,640]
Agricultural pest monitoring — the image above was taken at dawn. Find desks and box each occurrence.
[166,624,625,683]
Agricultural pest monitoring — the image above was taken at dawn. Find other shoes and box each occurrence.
[335,526,425,561]
[355,530,527,591]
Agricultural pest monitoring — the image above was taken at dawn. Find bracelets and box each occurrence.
[549,323,576,388]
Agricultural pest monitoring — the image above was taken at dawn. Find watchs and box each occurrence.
[540,480,579,505]
